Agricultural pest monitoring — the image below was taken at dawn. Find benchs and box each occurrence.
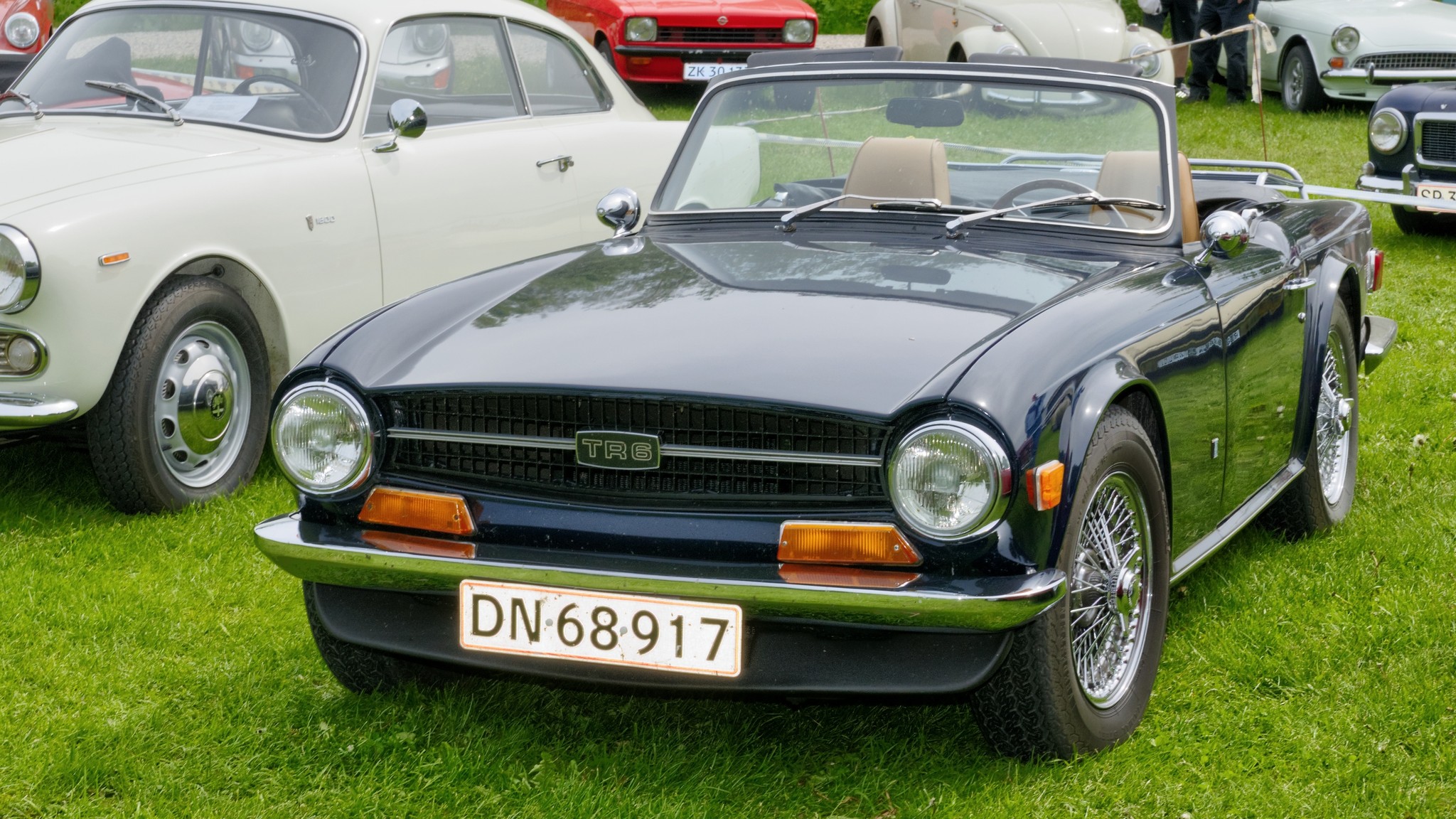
[334,104,591,134]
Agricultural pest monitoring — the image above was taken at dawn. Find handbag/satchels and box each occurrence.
[1139,0,1163,16]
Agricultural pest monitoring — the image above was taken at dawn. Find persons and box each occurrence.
[1142,0,1197,88]
[1186,0,1259,104]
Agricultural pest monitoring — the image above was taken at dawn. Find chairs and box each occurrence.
[1088,150,1199,244]
[837,136,952,209]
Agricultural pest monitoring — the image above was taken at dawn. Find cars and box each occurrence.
[0,1,818,515]
[252,46,1397,767]
[863,0,1174,121]
[1214,0,1456,238]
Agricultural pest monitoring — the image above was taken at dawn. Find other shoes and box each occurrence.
[1182,95,1206,105]
